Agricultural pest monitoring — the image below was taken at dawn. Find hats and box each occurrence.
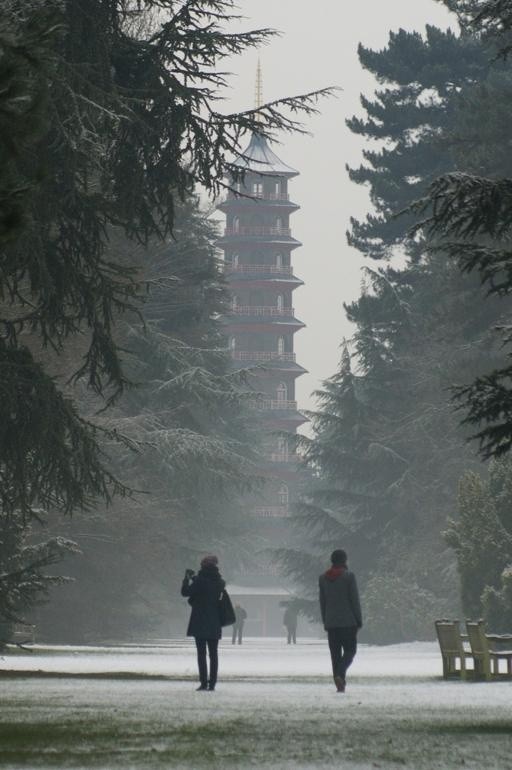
[200,555,219,569]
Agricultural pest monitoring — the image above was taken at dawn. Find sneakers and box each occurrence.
[196,686,216,692]
[335,676,347,693]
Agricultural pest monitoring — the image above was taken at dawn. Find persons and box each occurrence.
[282,606,300,643]
[317,550,363,693]
[231,603,247,646]
[179,555,226,692]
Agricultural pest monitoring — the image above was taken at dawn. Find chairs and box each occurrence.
[436,614,512,682]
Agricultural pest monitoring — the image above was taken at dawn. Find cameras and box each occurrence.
[187,569,195,577]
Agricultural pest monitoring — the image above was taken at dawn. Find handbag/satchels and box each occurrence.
[215,574,236,627]
